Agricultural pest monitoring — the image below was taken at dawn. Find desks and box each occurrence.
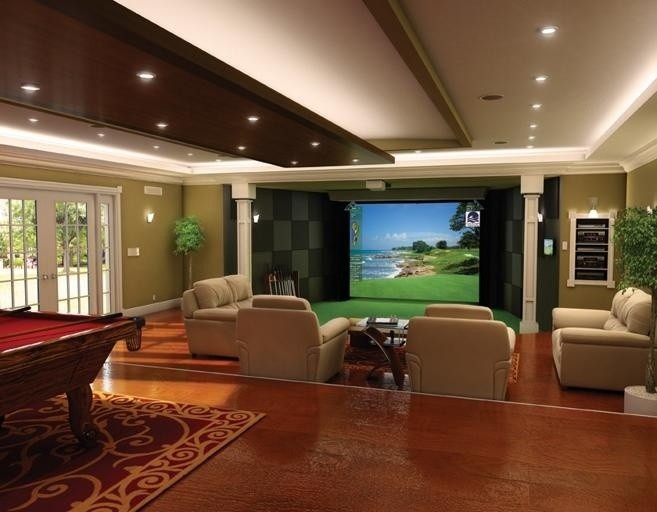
[0,305,146,448]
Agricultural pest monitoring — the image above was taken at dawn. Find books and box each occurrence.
[366,317,398,328]
[383,339,405,347]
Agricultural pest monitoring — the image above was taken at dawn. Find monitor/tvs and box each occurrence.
[346,199,483,304]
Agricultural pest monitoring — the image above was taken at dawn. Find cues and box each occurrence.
[0,305,31,317]
[0,312,122,339]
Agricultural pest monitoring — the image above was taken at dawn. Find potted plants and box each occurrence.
[610,204,657,417]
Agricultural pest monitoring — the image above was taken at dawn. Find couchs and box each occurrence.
[551,287,657,392]
[180,272,253,360]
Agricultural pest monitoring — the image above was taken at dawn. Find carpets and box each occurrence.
[1,389,266,512]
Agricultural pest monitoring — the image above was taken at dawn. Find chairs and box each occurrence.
[403,304,517,402]
[235,294,352,383]
